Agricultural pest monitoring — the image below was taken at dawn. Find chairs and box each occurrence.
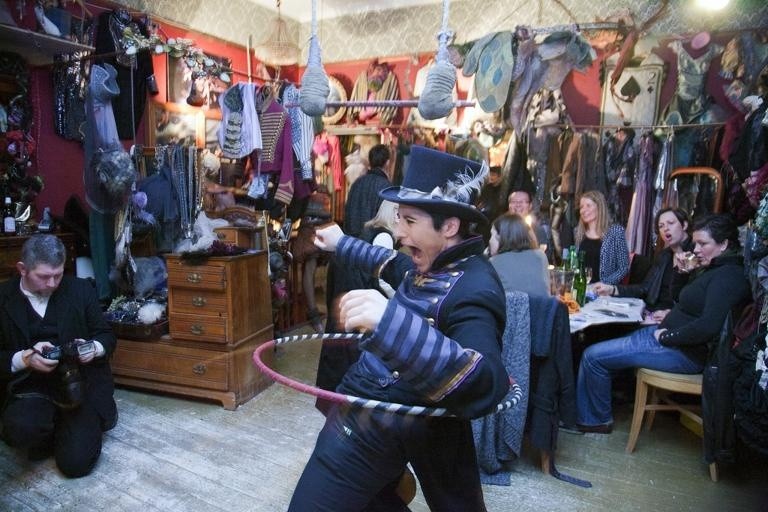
[504,290,567,473]
[626,366,719,484]
[619,252,637,285]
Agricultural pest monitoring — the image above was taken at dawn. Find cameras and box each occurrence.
[41,341,97,406]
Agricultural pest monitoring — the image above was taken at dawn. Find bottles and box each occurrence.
[570,245,577,278]
[576,250,588,305]
[558,245,569,273]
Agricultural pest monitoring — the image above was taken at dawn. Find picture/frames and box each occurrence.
[320,76,347,125]
[147,97,206,156]
[166,46,233,121]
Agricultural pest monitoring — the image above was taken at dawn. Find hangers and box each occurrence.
[561,125,676,145]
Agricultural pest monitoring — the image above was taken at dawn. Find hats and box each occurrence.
[310,77,348,124]
[378,145,491,224]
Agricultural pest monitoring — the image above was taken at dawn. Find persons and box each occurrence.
[0,233,119,480]
[339,143,393,234]
[509,189,549,259]
[656,32,725,124]
[314,198,406,421]
[574,214,751,434]
[287,144,512,512]
[574,190,631,285]
[487,212,554,298]
[583,206,701,343]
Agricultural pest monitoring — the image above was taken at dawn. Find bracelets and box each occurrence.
[610,285,615,297]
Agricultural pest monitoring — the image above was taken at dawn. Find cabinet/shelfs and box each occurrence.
[0,231,78,287]
[111,227,275,411]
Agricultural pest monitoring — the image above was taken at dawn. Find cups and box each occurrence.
[552,266,573,298]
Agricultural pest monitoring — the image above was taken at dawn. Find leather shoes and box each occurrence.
[557,424,613,433]
